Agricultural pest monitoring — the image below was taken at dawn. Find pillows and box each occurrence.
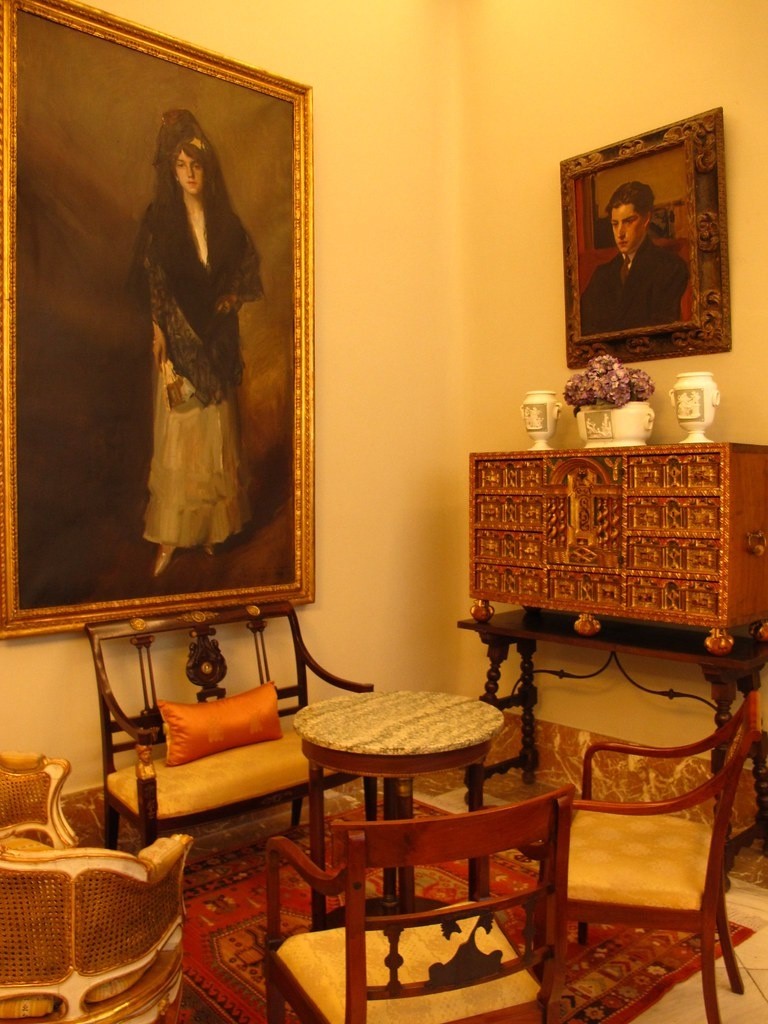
[155,679,283,767]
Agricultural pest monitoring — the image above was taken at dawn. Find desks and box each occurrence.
[457,607,768,894]
[292,689,506,932]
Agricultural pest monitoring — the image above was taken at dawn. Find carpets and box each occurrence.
[176,794,756,1024]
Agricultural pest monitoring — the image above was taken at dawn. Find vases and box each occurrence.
[576,402,655,448]
[668,371,721,442]
[520,390,562,450]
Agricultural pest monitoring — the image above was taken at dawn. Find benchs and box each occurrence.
[85,601,377,849]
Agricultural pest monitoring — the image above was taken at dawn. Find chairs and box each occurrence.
[0,751,196,1024]
[531,690,761,1024]
[265,783,577,1024]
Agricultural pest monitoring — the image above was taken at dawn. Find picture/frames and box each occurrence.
[559,106,732,369]
[0,0,317,640]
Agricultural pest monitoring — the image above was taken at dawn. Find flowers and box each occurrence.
[563,355,655,419]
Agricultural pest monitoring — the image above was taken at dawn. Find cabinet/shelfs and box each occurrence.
[469,442,768,655]
